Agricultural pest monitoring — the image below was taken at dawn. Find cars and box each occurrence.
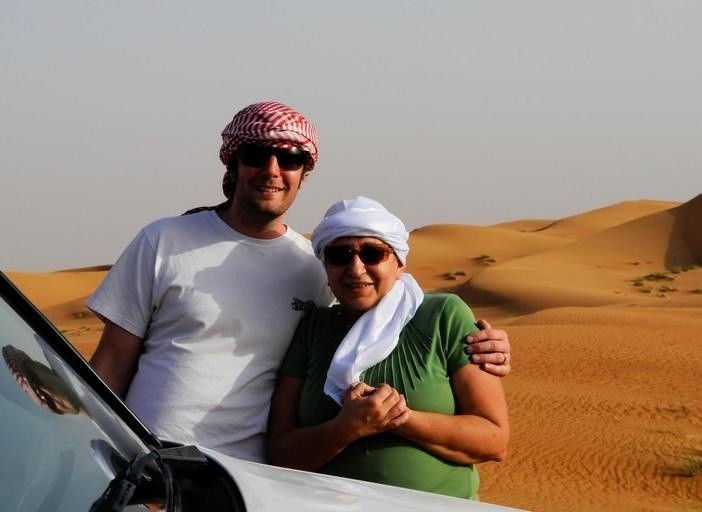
[1,272,529,512]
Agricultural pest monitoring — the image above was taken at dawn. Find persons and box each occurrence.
[2,327,139,478]
[91,102,511,463]
[269,197,509,498]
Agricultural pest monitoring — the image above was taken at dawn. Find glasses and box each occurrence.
[324,243,395,267]
[236,144,307,172]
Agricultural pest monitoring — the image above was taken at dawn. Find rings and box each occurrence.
[502,351,507,364]
